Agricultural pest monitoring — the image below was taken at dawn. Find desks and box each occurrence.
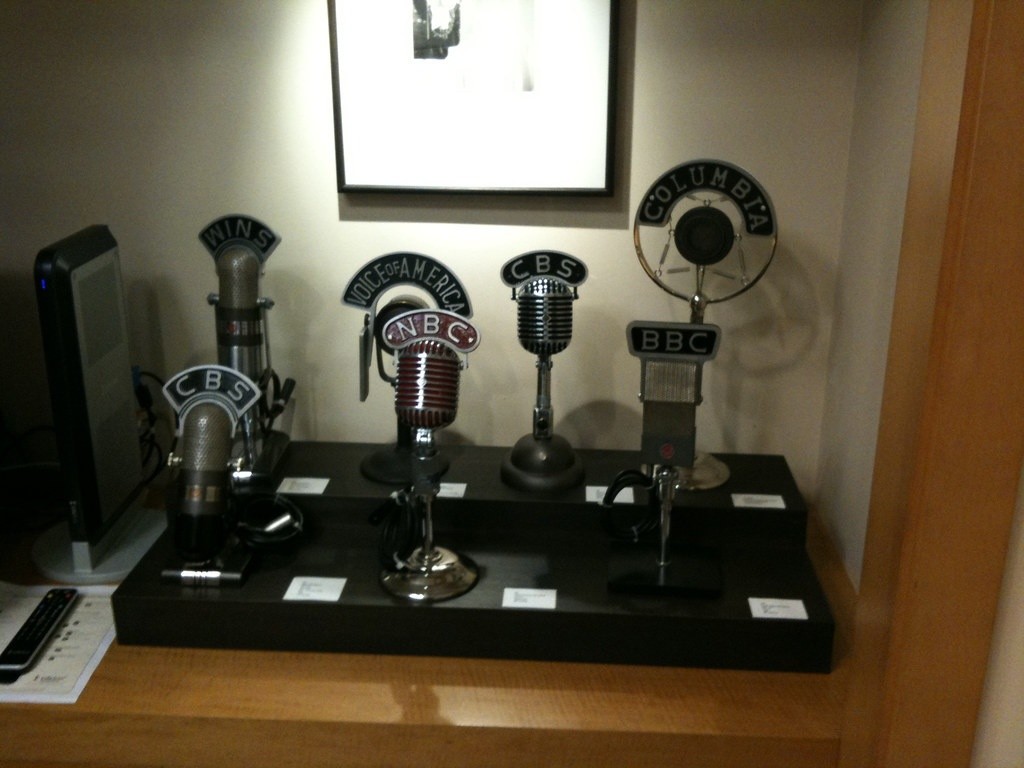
[0,485,858,768]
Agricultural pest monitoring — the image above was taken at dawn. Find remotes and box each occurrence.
[0,588,78,671]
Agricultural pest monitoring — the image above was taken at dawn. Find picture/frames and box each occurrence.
[328,0,618,198]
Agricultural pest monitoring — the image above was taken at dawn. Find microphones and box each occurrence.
[159,215,296,583]
[341,252,481,434]
[503,251,588,358]
[605,159,778,511]
